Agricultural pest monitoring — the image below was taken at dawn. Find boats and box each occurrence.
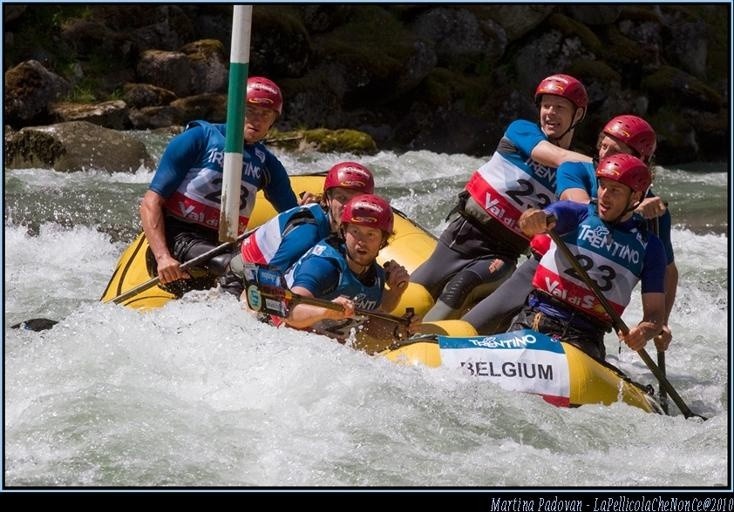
[97,173,669,420]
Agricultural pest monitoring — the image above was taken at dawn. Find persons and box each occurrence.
[407,74,598,323]
[505,153,667,361]
[257,194,410,329]
[460,115,679,352]
[214,162,374,300]
[140,77,300,297]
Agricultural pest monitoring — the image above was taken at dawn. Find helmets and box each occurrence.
[323,162,375,195]
[596,153,653,195]
[338,192,395,238]
[598,114,658,161]
[535,74,589,122]
[245,76,283,117]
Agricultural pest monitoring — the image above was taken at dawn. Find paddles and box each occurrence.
[241,263,410,327]
[12,225,260,332]
